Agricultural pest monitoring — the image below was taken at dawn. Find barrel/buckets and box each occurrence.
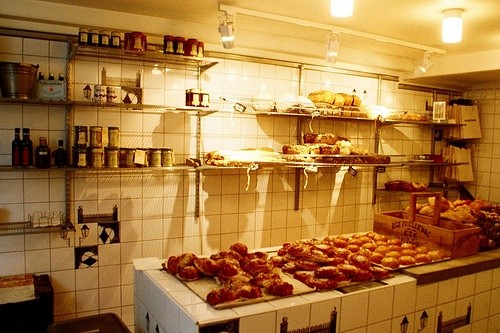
[0,61,40,101]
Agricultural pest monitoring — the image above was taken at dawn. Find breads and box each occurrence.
[385,180,428,193]
[163,232,444,305]
[406,196,500,249]
[282,132,389,164]
[303,91,365,117]
[384,111,428,121]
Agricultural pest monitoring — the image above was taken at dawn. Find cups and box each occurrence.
[108,86,122,103]
[94,85,107,103]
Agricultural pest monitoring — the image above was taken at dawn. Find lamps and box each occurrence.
[219,13,236,50]
[416,51,436,77]
[442,7,464,43]
[326,34,340,63]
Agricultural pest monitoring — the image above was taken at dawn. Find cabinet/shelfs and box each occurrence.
[0,28,469,237]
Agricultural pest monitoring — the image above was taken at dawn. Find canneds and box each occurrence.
[93,84,119,103]
[78,26,121,49]
[71,125,173,167]
[185,88,210,108]
[163,35,204,58]
[124,31,147,53]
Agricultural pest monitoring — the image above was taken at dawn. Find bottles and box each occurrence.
[52,140,67,168]
[73,124,174,167]
[11,128,21,167]
[35,136,51,169]
[37,71,45,80]
[21,128,34,167]
[77,26,205,57]
[47,72,55,80]
[186,89,209,108]
[58,72,65,81]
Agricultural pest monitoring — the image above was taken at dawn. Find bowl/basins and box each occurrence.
[384,180,407,191]
[414,154,432,160]
